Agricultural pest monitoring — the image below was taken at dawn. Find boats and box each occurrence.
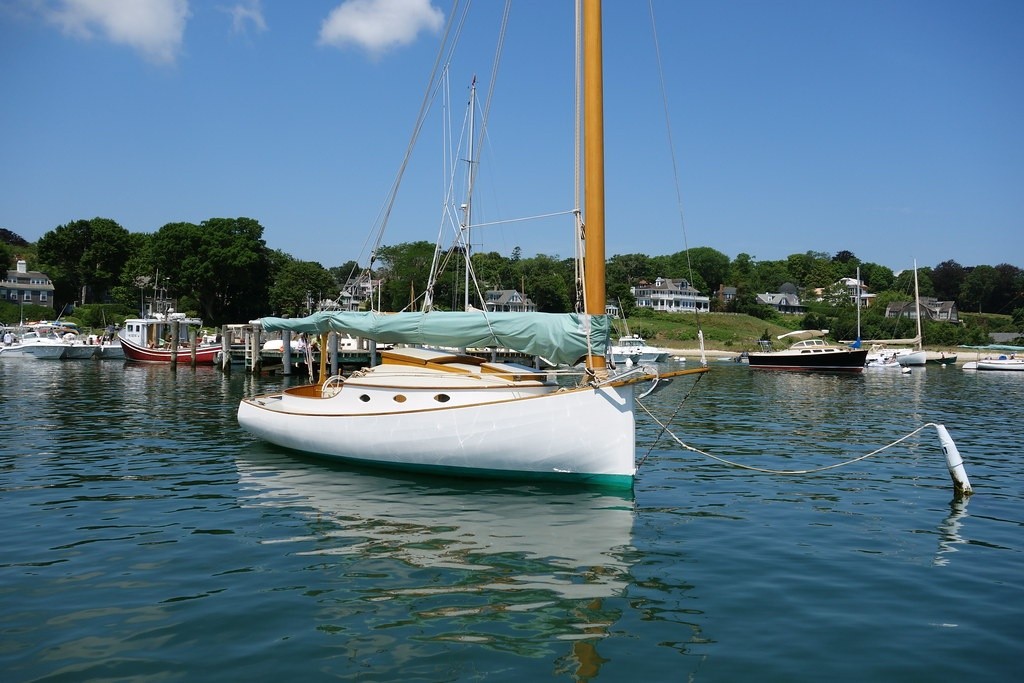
[607,296,670,367]
[116,267,224,365]
[0,302,125,360]
[925,348,959,364]
[962,352,1024,372]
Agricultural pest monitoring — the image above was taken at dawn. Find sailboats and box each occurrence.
[868,258,928,367]
[238,0,656,498]
[740,266,868,373]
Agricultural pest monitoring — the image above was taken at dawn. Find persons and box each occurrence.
[108,323,114,345]
[941,352,944,358]
[4,331,12,346]
